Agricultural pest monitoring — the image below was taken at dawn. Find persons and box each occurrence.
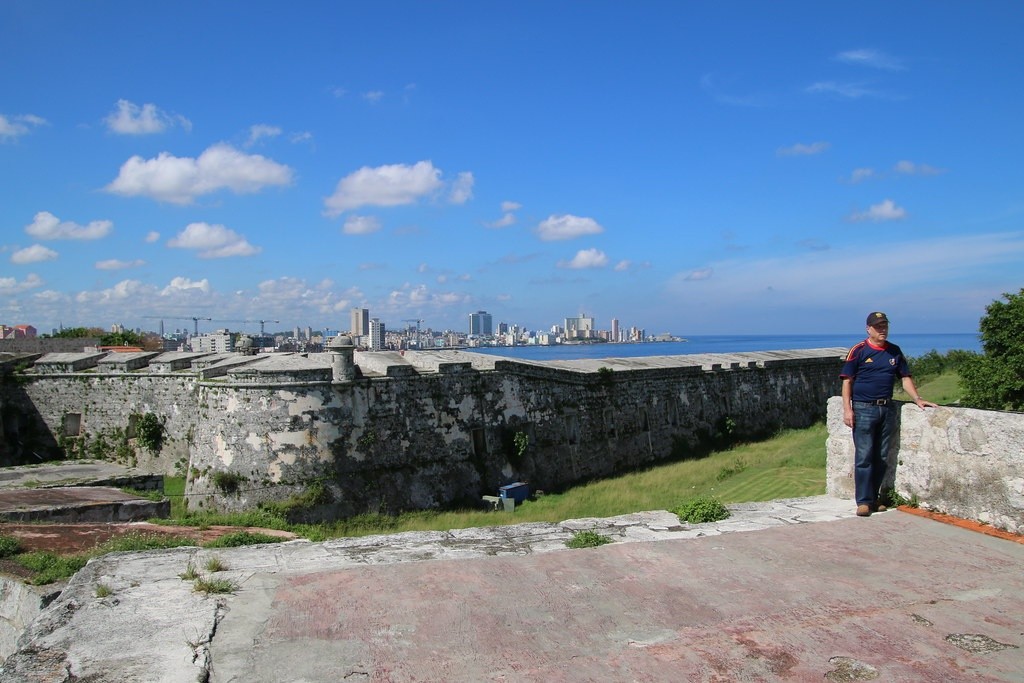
[839,312,938,516]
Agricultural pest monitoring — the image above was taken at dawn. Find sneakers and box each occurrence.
[857,503,870,516]
[871,503,887,511]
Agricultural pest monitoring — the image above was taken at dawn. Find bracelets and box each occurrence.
[912,396,922,403]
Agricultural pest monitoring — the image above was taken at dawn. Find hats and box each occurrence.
[866,311,890,326]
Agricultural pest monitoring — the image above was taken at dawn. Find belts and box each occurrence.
[867,399,893,405]
